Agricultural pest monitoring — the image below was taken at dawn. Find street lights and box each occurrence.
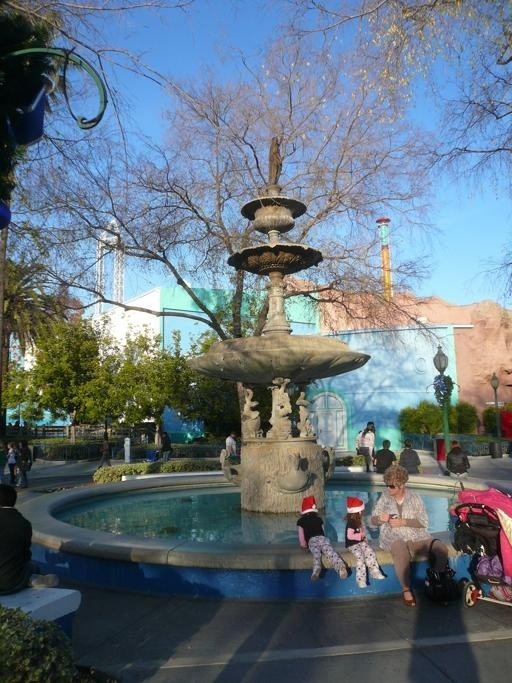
[1,41,116,152]
[490,371,502,440]
[432,343,453,458]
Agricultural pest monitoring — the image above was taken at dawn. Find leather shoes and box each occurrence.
[400,589,417,608]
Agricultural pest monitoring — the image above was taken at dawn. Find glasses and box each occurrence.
[386,484,398,489]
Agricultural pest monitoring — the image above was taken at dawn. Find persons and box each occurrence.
[358,421,376,472]
[445,440,470,478]
[344,498,385,589]
[98,437,111,469]
[372,464,448,608]
[321,450,330,476]
[399,438,422,474]
[0,484,32,598]
[226,431,238,457]
[374,440,395,471]
[0,419,34,489]
[155,430,174,461]
[501,402,512,463]
[296,495,348,581]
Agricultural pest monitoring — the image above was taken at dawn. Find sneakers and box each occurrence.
[30,573,59,588]
[311,565,322,581]
[356,580,367,589]
[371,573,386,580]
[339,563,349,580]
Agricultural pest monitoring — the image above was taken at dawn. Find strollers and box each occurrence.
[443,477,512,615]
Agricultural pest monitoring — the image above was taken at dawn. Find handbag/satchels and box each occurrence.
[424,568,458,605]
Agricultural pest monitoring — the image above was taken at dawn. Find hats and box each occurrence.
[345,495,365,514]
[300,495,319,515]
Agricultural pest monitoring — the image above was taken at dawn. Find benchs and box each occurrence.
[0,586,82,643]
